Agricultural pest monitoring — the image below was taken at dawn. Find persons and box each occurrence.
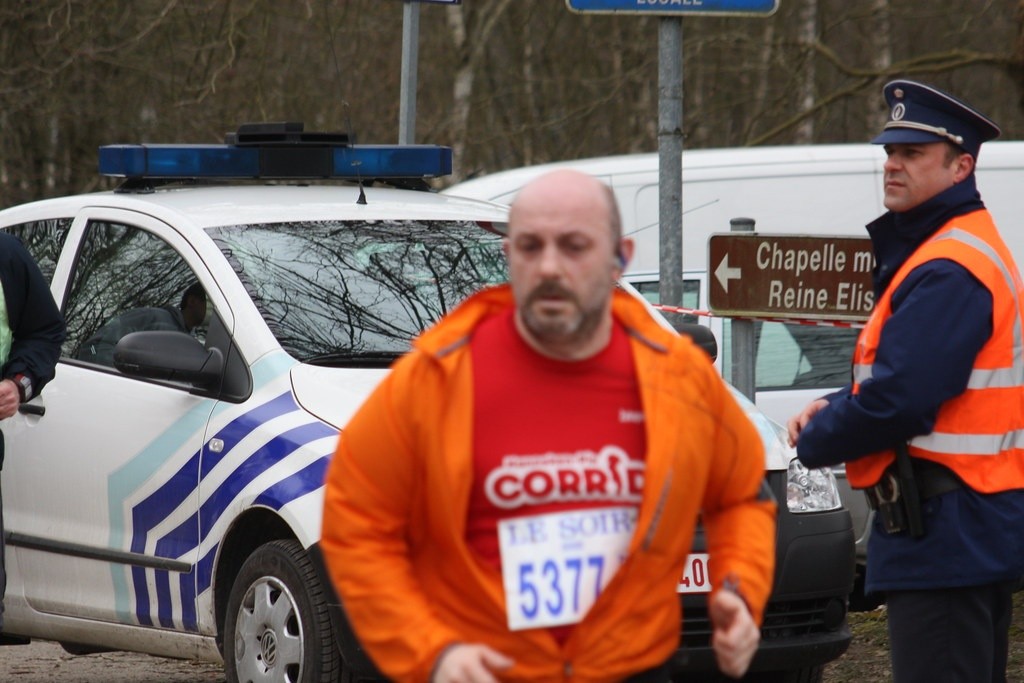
[321,166,778,683]
[787,81,1024,683]
[0,233,62,649]
[178,283,211,335]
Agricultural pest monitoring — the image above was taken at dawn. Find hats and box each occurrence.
[870,80,1002,161]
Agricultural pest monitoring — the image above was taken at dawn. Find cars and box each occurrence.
[2,122,857,683]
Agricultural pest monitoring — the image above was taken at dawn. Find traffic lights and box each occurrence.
[707,230,881,322]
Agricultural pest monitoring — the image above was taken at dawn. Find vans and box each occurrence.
[410,139,1024,613]
